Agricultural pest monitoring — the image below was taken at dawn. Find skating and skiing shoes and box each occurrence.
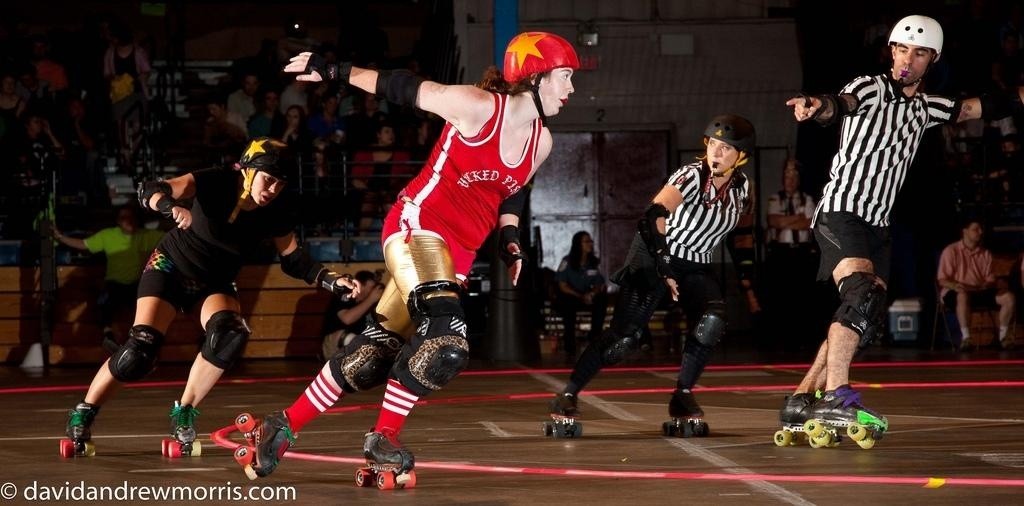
[234,410,299,481]
[542,392,582,439]
[59,398,101,458]
[803,384,889,450]
[662,385,710,439]
[354,426,417,491]
[773,393,844,447]
[160,400,202,458]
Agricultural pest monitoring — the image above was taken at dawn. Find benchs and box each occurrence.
[549,287,726,353]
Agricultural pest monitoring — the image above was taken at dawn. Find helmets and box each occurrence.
[238,135,293,182]
[887,15,944,64]
[502,31,581,84]
[703,114,756,158]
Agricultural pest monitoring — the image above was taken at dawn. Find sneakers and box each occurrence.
[960,337,978,354]
[996,336,1015,354]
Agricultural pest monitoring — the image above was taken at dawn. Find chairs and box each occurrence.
[930,266,1007,361]
[1006,261,1023,345]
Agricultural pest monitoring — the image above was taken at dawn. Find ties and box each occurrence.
[788,196,800,243]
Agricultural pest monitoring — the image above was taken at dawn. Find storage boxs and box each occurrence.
[888,298,922,344]
